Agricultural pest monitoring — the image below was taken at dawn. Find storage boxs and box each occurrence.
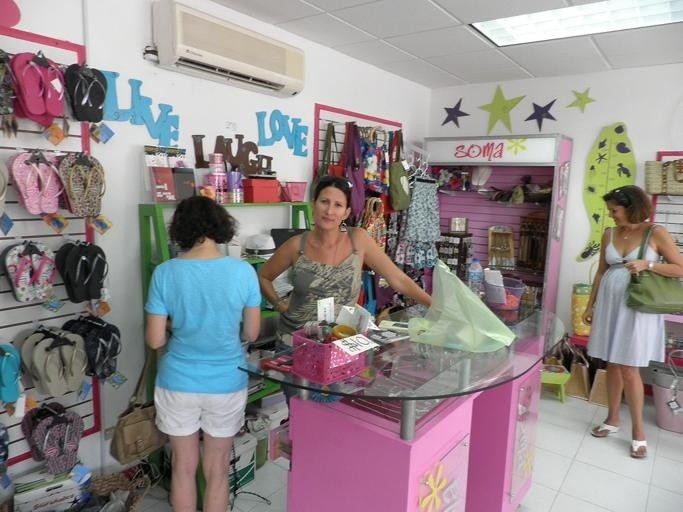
[242,178,279,203]
[281,180,307,201]
[163,435,257,497]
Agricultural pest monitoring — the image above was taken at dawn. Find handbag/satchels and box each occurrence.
[644,159,682,195]
[626,266,683,313]
[571,284,597,337]
[544,338,612,405]
[110,403,169,464]
[649,350,682,432]
[312,122,411,252]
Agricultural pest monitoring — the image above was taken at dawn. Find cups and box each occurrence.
[216,243,226,256]
[226,245,241,258]
[206,153,245,204]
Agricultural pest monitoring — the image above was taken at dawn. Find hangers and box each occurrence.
[392,152,440,190]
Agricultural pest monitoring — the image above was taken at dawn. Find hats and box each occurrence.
[243,236,276,262]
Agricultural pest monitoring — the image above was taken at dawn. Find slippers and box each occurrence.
[0,317,122,403]
[591,422,620,437]
[630,439,647,458]
[0,150,106,218]
[22,403,84,474]
[0,49,107,123]
[5,241,109,303]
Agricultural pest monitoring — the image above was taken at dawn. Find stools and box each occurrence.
[541,365,571,403]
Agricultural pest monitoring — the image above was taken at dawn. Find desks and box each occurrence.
[239,305,565,512]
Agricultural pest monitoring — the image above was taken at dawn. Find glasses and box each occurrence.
[614,190,630,205]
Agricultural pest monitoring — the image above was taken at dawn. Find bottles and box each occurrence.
[467,257,484,297]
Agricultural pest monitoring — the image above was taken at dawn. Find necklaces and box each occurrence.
[624,232,632,240]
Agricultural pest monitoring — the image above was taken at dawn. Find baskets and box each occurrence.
[485,277,527,311]
[292,327,365,385]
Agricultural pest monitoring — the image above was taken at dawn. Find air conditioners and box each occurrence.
[146,0,305,98]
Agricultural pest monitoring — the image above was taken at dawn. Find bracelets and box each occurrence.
[272,299,286,310]
[647,260,653,273]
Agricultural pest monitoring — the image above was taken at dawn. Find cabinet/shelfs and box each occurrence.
[424,135,573,350]
[140,202,313,509]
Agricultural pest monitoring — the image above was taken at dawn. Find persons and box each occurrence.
[143,195,262,511]
[257,176,432,408]
[581,184,683,459]
